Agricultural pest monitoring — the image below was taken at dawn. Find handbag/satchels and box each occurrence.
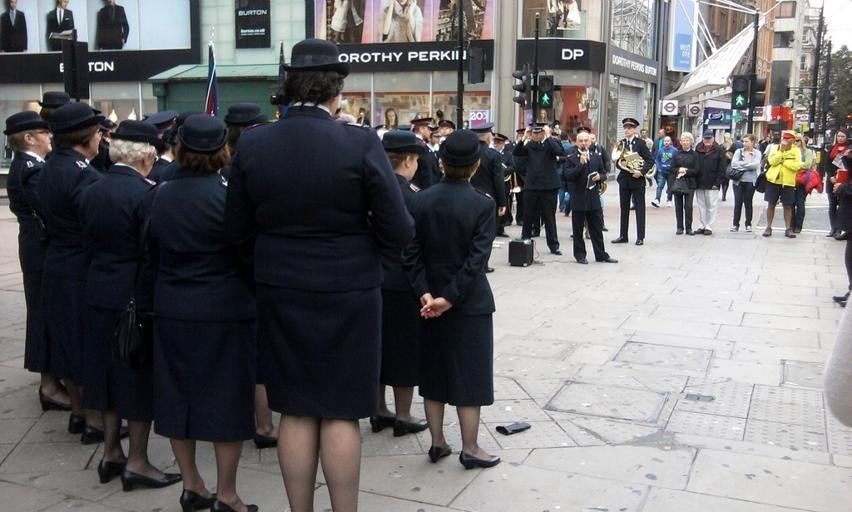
[725,149,744,181]
[670,167,690,195]
[755,171,766,192]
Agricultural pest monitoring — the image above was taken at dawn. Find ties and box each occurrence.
[627,140,630,149]
[10,9,15,25]
[59,9,61,24]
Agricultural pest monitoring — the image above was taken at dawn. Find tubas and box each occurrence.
[612,141,656,181]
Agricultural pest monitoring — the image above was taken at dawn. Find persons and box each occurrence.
[47,0,76,51]
[443,0,487,40]
[0,0,28,53]
[547,0,582,30]
[833,146,852,308]
[5,39,500,512]
[499,121,618,265]
[331,0,362,43]
[96,0,130,50]
[382,0,423,42]
[650,128,851,241]
[610,118,654,246]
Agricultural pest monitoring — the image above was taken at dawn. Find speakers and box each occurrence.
[509,239,533,267]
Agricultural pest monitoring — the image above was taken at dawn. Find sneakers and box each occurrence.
[651,201,660,208]
[730,226,738,232]
[745,225,752,232]
[762,227,772,236]
[785,229,796,238]
[666,202,672,207]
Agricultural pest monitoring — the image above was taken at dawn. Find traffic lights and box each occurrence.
[511,63,532,111]
[538,73,554,109]
[732,74,748,111]
[752,74,767,108]
[823,90,835,112]
[845,100,852,120]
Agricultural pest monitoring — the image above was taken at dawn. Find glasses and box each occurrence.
[795,140,801,142]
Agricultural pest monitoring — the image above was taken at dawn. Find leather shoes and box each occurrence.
[500,231,509,237]
[826,230,847,240]
[676,228,712,235]
[38,387,278,511]
[459,451,500,469]
[611,237,628,243]
[636,238,643,244]
[551,249,561,255]
[487,267,494,272]
[370,415,395,432]
[393,418,428,436]
[832,291,849,301]
[428,446,451,463]
[570,228,618,264]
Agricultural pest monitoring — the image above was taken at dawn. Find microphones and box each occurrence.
[581,145,588,163]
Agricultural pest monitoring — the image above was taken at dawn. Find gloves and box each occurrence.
[495,422,531,435]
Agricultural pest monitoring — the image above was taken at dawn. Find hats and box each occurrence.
[783,133,795,139]
[703,130,714,138]
[438,129,487,165]
[622,118,640,128]
[282,38,350,76]
[410,117,548,141]
[382,131,428,152]
[577,127,591,133]
[3,91,267,151]
